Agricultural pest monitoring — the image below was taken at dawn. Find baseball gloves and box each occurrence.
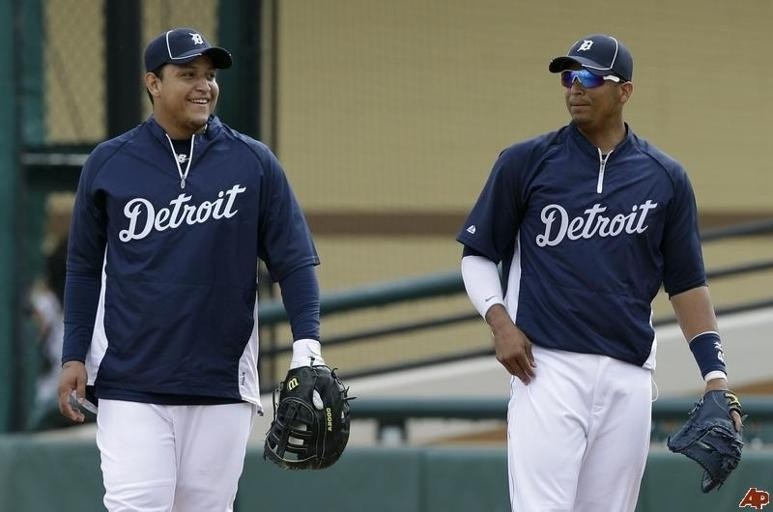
[667,389,744,492]
[263,365,351,470]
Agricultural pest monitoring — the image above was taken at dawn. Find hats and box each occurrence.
[548,33,633,86]
[143,26,234,74]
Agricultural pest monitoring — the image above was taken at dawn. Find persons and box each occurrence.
[455,36,742,511]
[58,27,324,511]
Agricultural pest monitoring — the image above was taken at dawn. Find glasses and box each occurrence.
[560,69,621,88]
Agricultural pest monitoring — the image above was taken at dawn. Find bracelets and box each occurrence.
[686,322,729,383]
